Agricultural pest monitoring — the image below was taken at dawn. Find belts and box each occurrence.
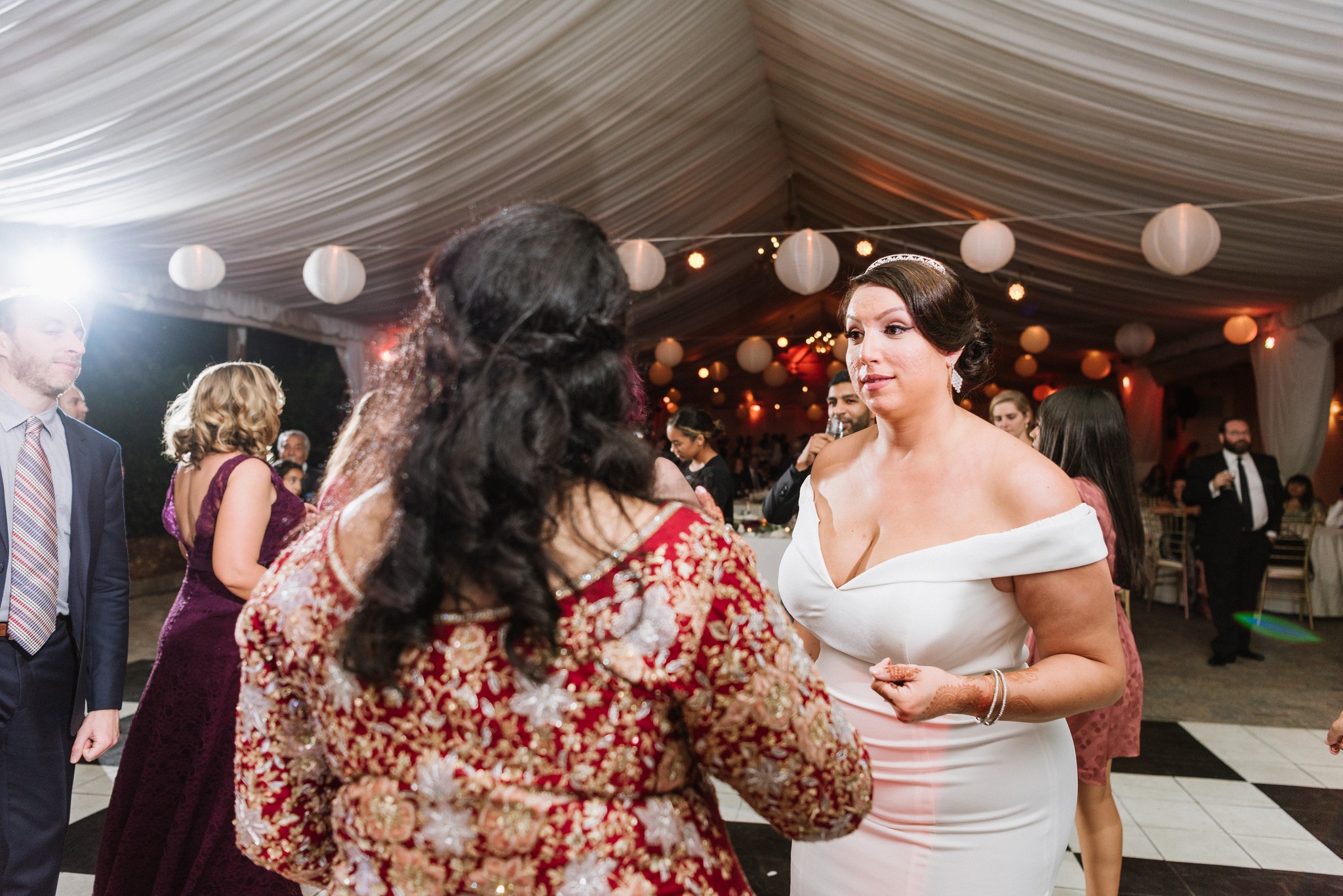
[0,623,14,639]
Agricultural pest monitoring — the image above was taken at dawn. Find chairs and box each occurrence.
[1255,514,1319,632]
[1135,491,1199,621]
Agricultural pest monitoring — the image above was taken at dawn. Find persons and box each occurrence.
[57,385,89,423]
[233,198,877,896]
[1182,416,1286,667]
[987,387,1031,447]
[274,428,310,497]
[761,369,877,526]
[768,247,1126,896]
[647,421,812,501]
[663,404,736,530]
[1144,439,1329,619]
[0,256,133,896]
[1030,382,1143,896]
[94,361,309,896]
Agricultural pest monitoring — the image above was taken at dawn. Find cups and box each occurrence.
[824,418,844,441]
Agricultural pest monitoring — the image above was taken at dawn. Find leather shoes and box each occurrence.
[1237,649,1264,663]
[1207,652,1235,667]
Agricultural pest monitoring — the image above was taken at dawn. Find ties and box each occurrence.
[6,412,64,658]
[1236,457,1255,530]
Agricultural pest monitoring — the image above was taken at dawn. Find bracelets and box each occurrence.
[975,669,999,725]
[988,669,1007,725]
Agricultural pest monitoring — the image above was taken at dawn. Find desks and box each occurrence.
[1204,509,1343,627]
